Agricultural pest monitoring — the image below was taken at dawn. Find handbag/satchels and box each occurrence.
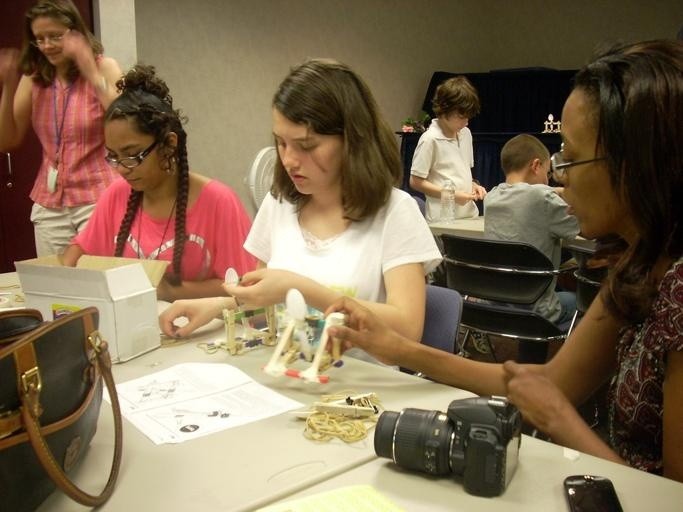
[0,305,124,512]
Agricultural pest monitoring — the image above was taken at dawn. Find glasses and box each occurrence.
[549,142,608,180]
[104,136,162,170]
[30,27,71,48]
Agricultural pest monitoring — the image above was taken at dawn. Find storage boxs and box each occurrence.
[11,254,176,366]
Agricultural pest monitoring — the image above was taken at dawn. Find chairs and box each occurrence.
[401,282,464,378]
[439,232,579,361]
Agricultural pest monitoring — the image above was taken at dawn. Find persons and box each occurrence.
[58,63,257,302]
[323,40,682,482]
[154,58,442,372]
[0,0,124,259]
[480,133,584,324]
[408,77,494,356]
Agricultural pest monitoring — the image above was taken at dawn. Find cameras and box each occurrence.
[374,395,522,497]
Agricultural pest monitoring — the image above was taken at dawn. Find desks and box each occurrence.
[251,429,683,509]
[0,264,481,509]
[430,210,612,254]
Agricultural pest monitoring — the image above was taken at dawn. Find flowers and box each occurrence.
[397,114,427,135]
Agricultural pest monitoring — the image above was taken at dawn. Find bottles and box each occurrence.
[439,179,455,226]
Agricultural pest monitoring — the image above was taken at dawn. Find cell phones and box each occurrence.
[564,475,623,512]
